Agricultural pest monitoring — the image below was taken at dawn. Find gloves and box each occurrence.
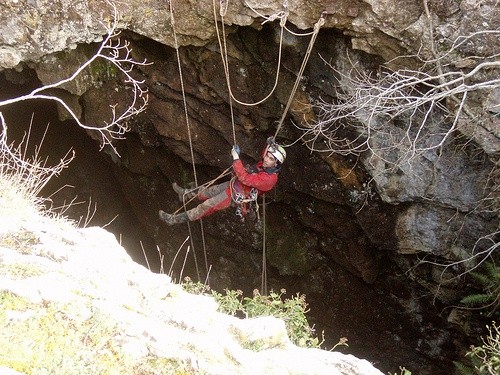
[231,145,240,160]
[267,136,275,146]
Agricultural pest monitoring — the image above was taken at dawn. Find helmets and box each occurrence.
[268,144,286,164]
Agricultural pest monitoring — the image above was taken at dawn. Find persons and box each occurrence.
[158,143,287,227]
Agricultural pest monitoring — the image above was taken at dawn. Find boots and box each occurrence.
[173,182,199,202]
[159,210,191,226]
